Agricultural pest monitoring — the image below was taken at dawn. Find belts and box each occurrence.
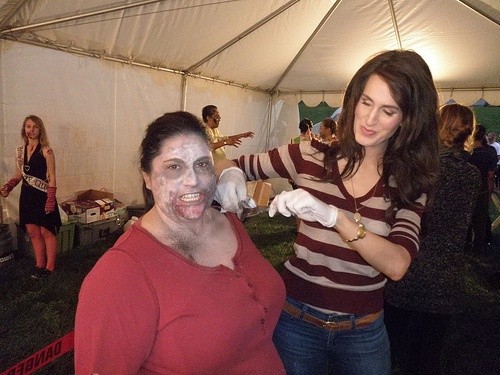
[283,302,384,332]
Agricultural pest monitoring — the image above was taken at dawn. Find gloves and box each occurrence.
[213,167,247,213]
[268,188,338,228]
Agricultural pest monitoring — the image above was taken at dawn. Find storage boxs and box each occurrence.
[96,196,115,218]
[77,188,112,201]
[76,198,128,249]
[16,221,74,255]
[253,180,272,207]
[63,201,100,223]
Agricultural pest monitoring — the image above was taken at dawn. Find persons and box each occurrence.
[379,103,485,375]
[288,118,319,236]
[466,123,497,251]
[486,130,500,189]
[201,106,254,214]
[304,119,339,146]
[0,115,62,281]
[72,108,288,375]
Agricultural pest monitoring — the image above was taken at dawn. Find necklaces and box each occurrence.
[213,49,438,375]
[351,168,386,221]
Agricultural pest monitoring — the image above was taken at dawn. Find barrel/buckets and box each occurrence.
[0,223,16,282]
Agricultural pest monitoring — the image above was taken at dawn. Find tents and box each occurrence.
[0,0,500,249]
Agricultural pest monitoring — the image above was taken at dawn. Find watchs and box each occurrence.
[344,221,366,242]
